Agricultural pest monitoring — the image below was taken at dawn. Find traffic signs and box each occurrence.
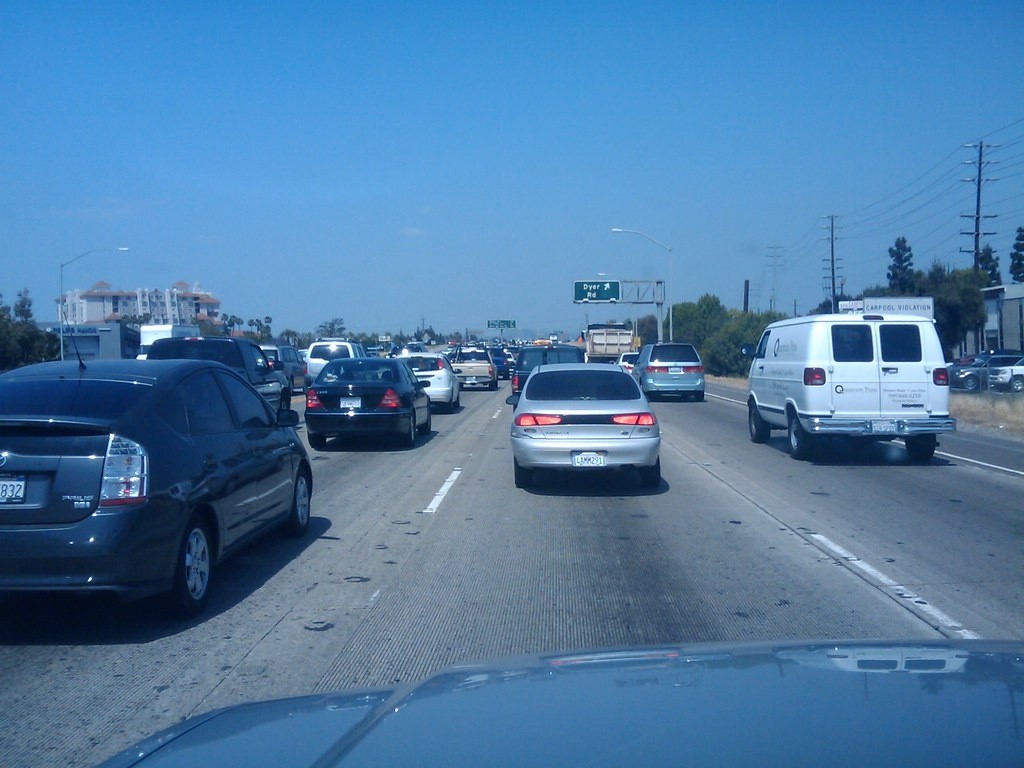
[573,279,622,304]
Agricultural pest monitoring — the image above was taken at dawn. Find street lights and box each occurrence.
[60,243,131,360]
[612,227,673,343]
[596,271,636,332]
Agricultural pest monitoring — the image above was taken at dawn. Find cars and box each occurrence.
[395,355,464,411]
[304,356,430,451]
[0,357,306,630]
[503,361,662,486]
[297,347,307,364]
[613,352,638,375]
[944,348,1024,392]
[364,333,563,382]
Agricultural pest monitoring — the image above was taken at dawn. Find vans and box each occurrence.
[257,344,308,393]
[304,337,368,383]
[741,311,958,461]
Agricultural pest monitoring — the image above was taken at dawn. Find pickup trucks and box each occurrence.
[447,347,501,392]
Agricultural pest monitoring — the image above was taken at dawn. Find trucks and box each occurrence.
[582,322,637,365]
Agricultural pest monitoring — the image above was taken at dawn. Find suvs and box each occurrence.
[148,338,294,422]
[508,344,586,413]
[627,341,708,404]
